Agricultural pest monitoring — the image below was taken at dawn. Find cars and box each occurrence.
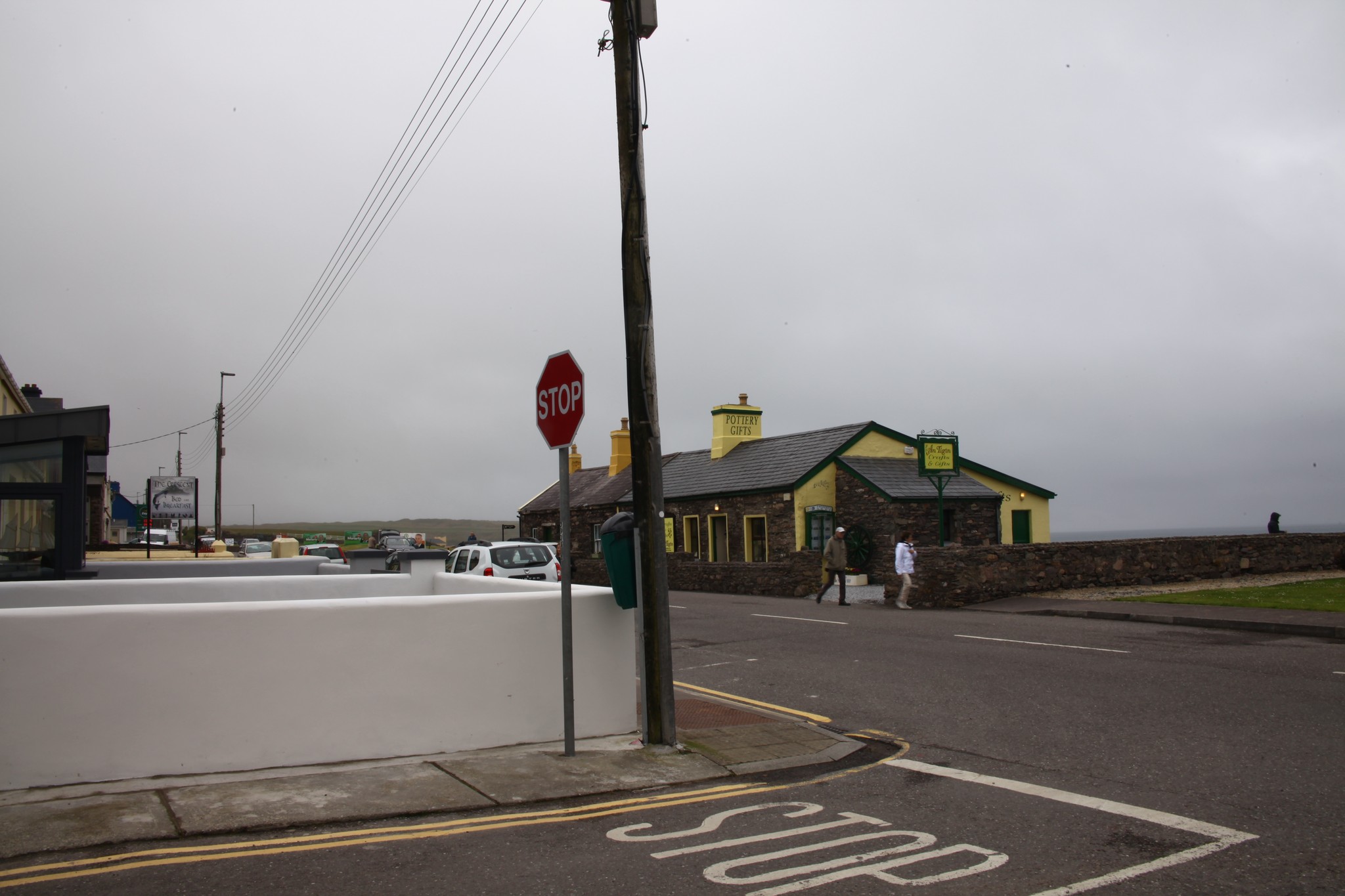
[237,541,272,558]
[119,538,141,544]
[385,551,400,571]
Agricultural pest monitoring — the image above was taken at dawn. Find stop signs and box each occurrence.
[536,349,585,450]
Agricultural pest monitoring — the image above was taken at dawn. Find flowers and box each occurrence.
[845,567,860,575]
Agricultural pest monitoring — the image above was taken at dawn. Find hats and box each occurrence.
[836,527,845,533]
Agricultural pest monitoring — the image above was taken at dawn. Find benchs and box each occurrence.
[500,554,536,566]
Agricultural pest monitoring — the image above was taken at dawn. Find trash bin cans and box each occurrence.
[600,511,637,611]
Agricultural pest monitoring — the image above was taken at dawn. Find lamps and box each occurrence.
[1020,492,1025,501]
[715,504,719,511]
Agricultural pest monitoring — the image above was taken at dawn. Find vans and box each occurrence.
[140,529,176,546]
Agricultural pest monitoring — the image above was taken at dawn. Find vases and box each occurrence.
[834,575,868,585]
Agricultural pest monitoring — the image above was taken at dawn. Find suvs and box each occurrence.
[299,544,347,565]
[376,529,417,554]
[445,536,562,584]
[190,530,216,550]
[240,538,273,549]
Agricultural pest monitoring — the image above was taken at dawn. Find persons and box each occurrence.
[1268,512,1287,533]
[369,535,377,549]
[361,533,369,543]
[895,532,918,611]
[412,534,425,549]
[814,527,852,606]
[381,539,384,543]
[468,532,477,541]
[317,535,326,543]
[555,543,576,579]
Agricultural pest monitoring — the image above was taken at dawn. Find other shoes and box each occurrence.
[896,602,913,610]
[816,596,821,604]
[838,601,851,606]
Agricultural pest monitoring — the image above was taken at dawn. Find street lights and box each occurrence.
[178,432,187,544]
[143,494,146,505]
[158,466,165,477]
[215,370,236,541]
[137,492,141,504]
